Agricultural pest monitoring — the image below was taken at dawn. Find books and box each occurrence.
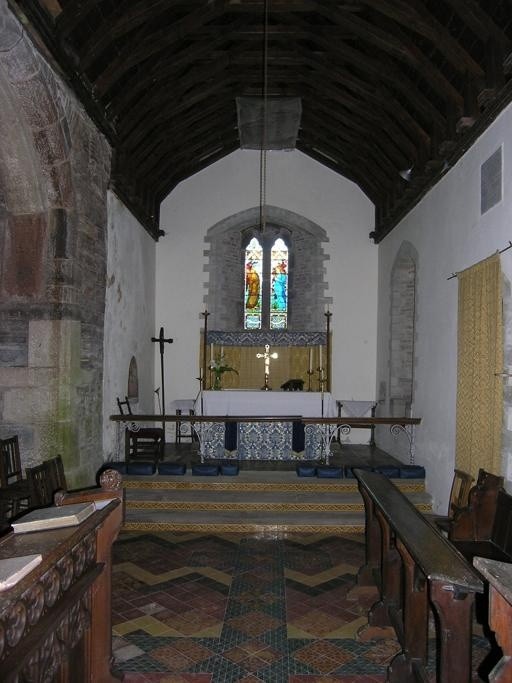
[10,500,96,534]
[0,552,43,592]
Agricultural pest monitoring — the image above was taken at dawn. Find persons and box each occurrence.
[273,265,287,310]
[246,262,260,309]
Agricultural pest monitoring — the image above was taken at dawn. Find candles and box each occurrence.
[210,343,226,361]
[309,345,323,372]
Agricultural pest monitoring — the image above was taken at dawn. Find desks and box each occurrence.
[200,391,331,461]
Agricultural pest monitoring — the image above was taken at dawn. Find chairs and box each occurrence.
[424,468,474,539]
[0,435,75,519]
[116,395,165,462]
